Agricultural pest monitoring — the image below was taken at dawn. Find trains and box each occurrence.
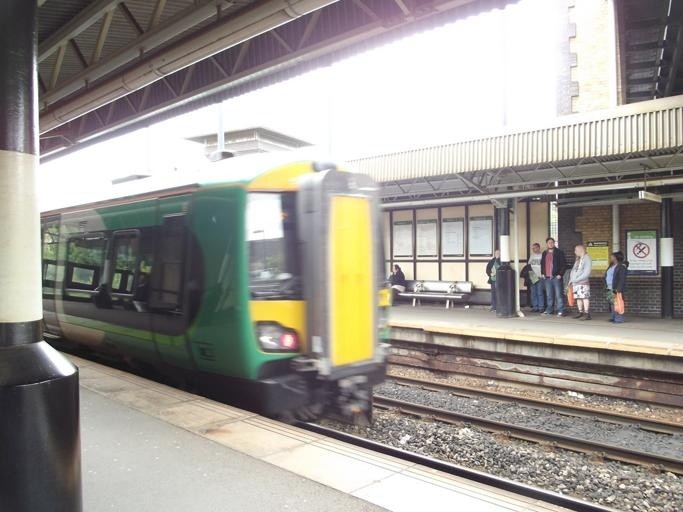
[41,150,399,431]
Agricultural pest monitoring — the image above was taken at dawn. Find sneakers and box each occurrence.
[541,311,553,316]
[608,317,622,323]
[557,312,566,317]
[532,308,545,313]
[580,313,591,321]
[573,313,583,318]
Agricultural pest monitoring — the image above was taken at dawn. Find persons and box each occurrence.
[604,251,629,323]
[387,265,406,307]
[487,238,592,320]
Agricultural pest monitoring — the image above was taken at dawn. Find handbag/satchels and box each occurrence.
[612,291,625,315]
[566,285,576,307]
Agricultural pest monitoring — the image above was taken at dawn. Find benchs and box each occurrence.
[397,280,472,307]
